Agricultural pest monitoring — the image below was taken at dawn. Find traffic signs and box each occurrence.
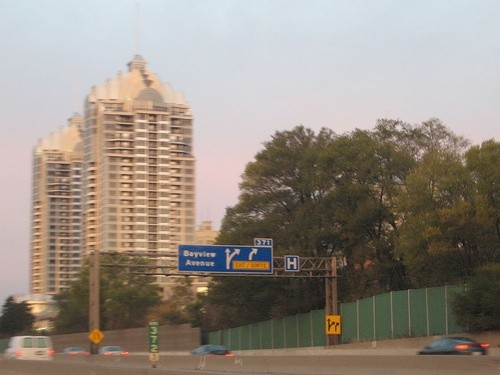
[178,244,273,274]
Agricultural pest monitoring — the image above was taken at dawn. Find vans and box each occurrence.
[3,335,57,361]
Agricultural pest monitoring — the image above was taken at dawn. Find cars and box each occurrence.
[61,345,92,356]
[193,344,234,359]
[98,345,129,356]
[418,336,489,356]
